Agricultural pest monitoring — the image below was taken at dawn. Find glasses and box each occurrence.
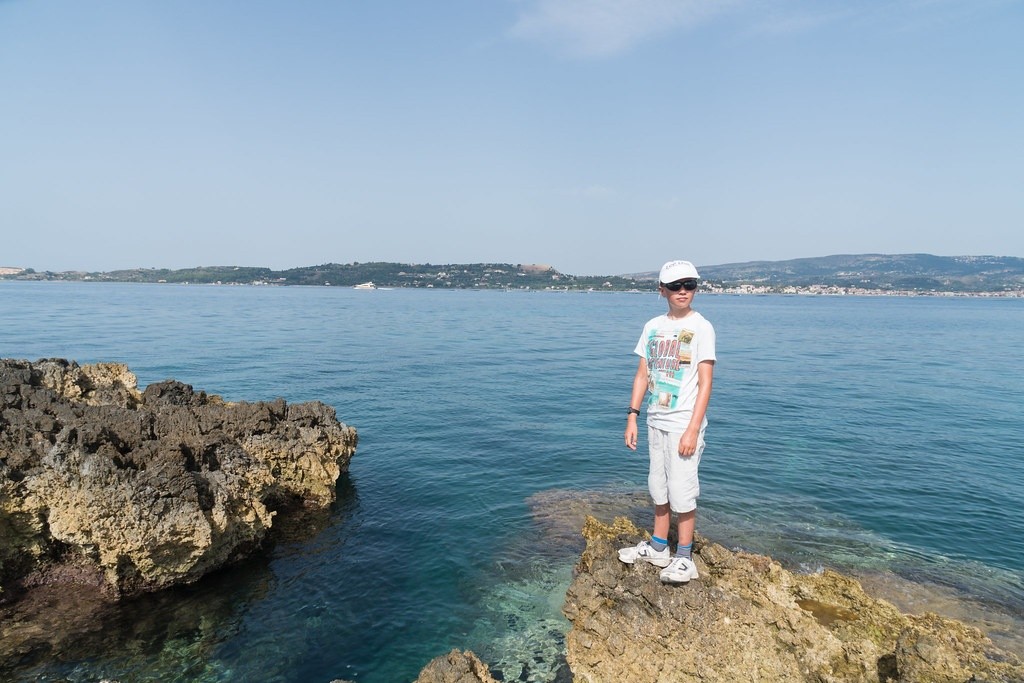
[666,280,697,291]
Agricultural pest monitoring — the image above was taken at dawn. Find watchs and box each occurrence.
[626,407,640,416]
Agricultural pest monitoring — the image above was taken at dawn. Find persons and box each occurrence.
[617,260,716,583]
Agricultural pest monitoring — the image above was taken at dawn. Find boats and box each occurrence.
[353,281,378,290]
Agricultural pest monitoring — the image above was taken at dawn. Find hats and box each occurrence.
[659,261,700,285]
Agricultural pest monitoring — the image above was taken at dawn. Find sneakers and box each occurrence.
[618,541,670,567]
[660,558,699,583]
